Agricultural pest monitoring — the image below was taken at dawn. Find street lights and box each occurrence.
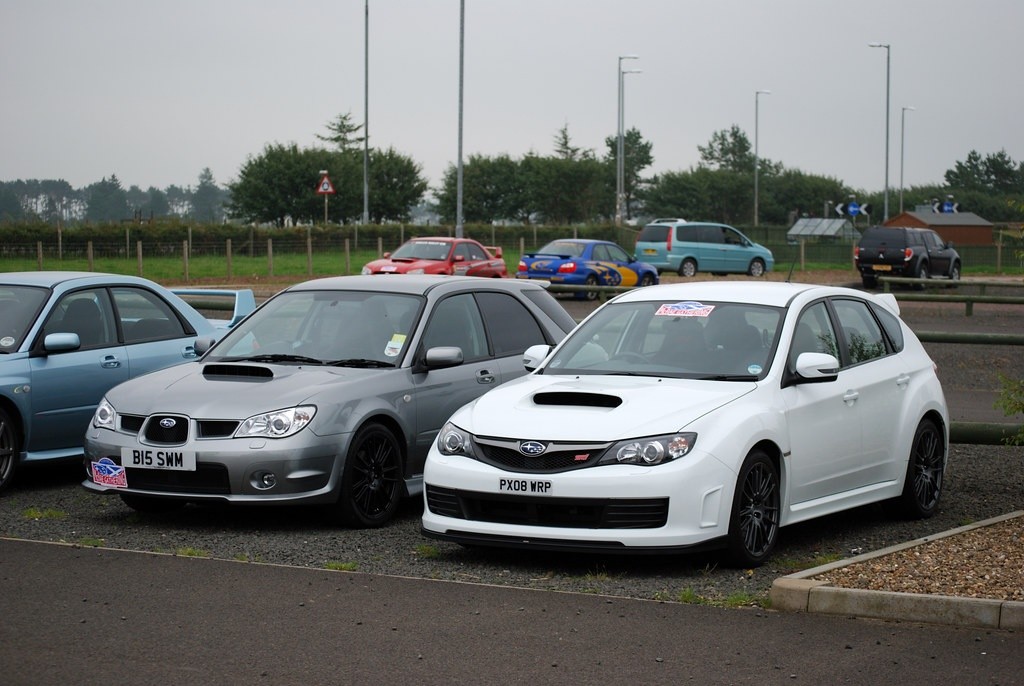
[752,87,772,229]
[866,43,891,223]
[615,55,639,221]
[897,108,917,215]
[620,69,646,209]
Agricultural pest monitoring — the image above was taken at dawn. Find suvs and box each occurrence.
[852,225,962,289]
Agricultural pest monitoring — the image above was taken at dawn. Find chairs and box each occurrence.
[0,300,28,340]
[58,298,103,346]
[335,308,472,365]
[656,319,864,376]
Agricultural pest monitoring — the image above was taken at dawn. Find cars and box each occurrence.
[82,273,607,527]
[362,236,507,280]
[0,269,260,498]
[418,278,953,570]
[634,218,775,277]
[513,238,658,301]
